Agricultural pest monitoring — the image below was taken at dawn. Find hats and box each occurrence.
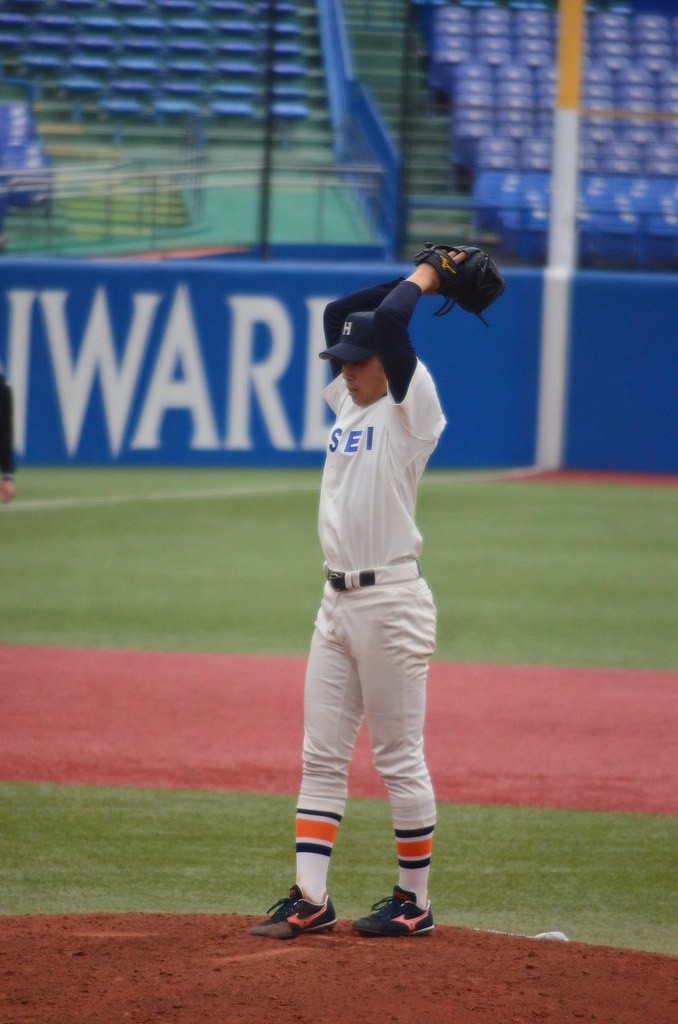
[317,309,386,363]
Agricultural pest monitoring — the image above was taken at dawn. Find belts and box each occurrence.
[323,562,421,592]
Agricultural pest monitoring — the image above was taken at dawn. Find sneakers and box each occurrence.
[350,884,434,936]
[248,884,338,939]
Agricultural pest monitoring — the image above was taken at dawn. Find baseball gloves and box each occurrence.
[411,241,506,331]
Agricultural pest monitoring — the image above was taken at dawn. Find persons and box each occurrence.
[0,367,25,514]
[247,238,504,942]
